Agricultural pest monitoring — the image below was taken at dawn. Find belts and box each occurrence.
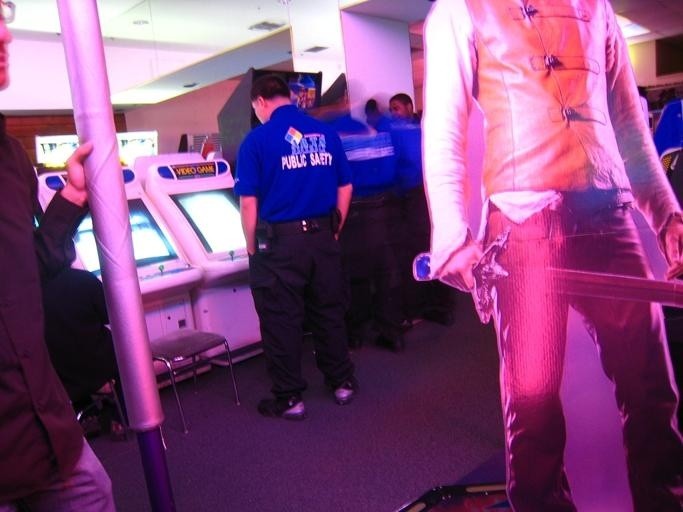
[267,217,333,233]
[352,199,388,207]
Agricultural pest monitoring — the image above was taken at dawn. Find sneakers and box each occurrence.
[258,310,455,420]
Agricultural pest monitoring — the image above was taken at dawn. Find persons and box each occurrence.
[0,0,117,511]
[418,0,683,512]
[38,267,129,434]
[227,74,455,421]
[640,87,683,200]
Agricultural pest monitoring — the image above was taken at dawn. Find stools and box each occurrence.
[74,381,131,441]
[150,327,242,434]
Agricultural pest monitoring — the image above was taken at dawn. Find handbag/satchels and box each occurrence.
[41,269,116,407]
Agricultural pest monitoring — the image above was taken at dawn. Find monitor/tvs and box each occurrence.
[73,198,179,277]
[169,187,246,254]
